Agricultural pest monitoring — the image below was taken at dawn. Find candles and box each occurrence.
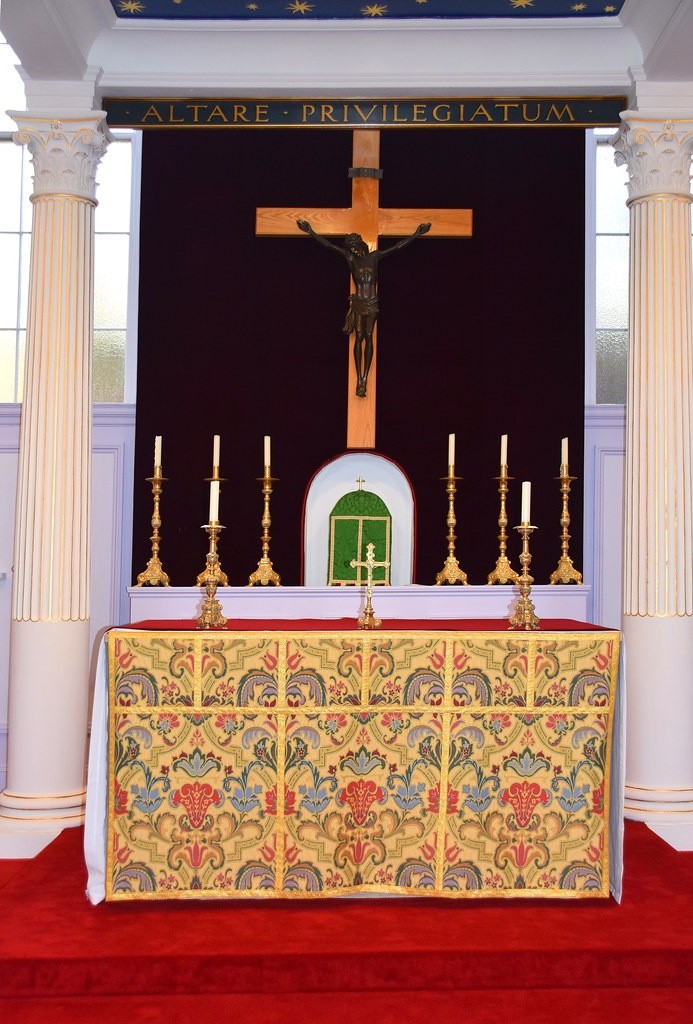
[154,436,162,466]
[214,435,221,466]
[520,481,531,522]
[448,433,455,465]
[500,434,508,465]
[264,435,271,465]
[561,437,568,464]
[209,480,220,520]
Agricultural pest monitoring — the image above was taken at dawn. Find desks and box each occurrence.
[84,618,627,903]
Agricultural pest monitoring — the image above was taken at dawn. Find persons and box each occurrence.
[296,220,431,396]
